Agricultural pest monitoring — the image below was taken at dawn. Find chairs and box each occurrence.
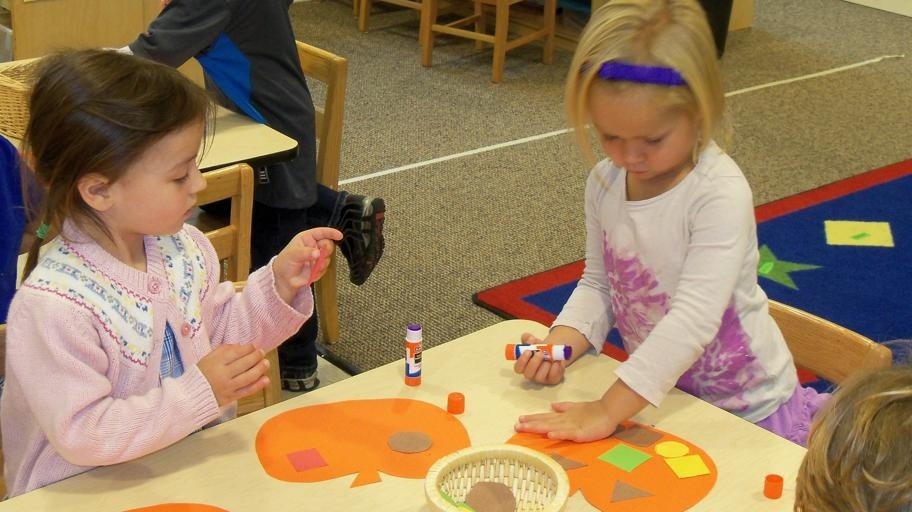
[2,324,11,500]
[768,298,893,387]
[196,162,254,282]
[354,0,556,83]
[295,40,348,345]
[229,282,283,416]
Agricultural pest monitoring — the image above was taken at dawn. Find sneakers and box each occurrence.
[334,193,386,286]
[280,365,320,392]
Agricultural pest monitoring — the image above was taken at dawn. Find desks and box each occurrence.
[1,320,809,512]
[0,100,299,173]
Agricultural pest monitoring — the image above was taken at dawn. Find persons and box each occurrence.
[1,45,349,492]
[789,338,911,511]
[104,1,387,393]
[505,2,832,453]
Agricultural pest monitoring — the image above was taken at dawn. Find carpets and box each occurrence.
[472,158,911,393]
